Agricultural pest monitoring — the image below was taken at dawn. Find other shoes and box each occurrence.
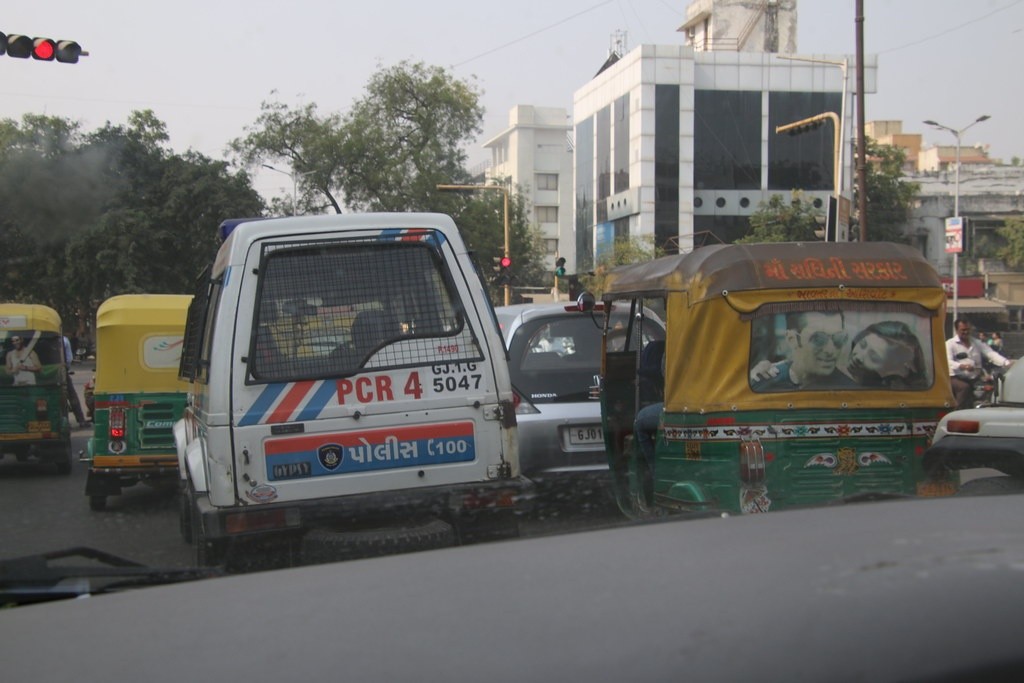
[643,468,655,480]
[80,422,90,428]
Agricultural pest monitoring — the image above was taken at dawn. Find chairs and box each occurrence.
[573,327,616,366]
[340,310,402,372]
[256,327,296,380]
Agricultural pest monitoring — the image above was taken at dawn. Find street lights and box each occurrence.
[922,110,991,336]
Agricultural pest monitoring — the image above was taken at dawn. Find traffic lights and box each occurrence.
[555,256,566,277]
[0,32,90,65]
[499,257,510,284]
[785,119,829,134]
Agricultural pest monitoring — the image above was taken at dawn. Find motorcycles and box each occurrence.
[1,300,73,475]
[65,369,96,422]
[570,238,958,520]
[80,288,200,516]
[951,351,1009,407]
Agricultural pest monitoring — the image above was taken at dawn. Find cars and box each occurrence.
[917,351,1024,489]
[0,544,170,611]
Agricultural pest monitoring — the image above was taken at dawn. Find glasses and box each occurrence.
[794,330,849,350]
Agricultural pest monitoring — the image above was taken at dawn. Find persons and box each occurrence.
[635,402,667,512]
[62,336,91,428]
[5,336,41,386]
[944,318,1013,409]
[750,308,929,391]
[978,330,1007,358]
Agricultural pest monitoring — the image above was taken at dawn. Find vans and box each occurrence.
[171,215,547,569]
[496,294,667,498]
